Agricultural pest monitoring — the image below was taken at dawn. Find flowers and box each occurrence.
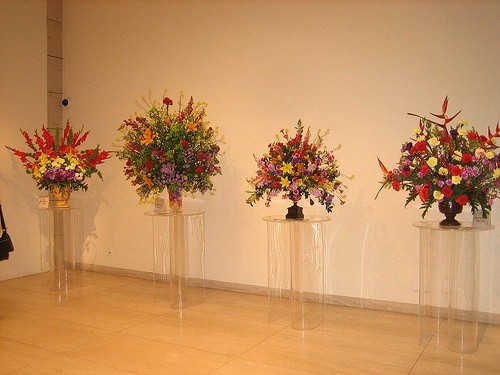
[245,119,355,214]
[4,118,113,193]
[113,88,227,203]
[373,95,500,218]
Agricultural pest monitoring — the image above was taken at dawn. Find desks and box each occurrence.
[35,202,91,292]
[412,220,495,354]
[263,215,331,330]
[143,209,206,308]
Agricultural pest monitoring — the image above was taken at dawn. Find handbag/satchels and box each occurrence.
[0,231,14,261]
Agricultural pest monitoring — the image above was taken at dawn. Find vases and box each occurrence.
[168,185,183,210]
[438,200,463,227]
[51,184,71,208]
[286,202,304,219]
